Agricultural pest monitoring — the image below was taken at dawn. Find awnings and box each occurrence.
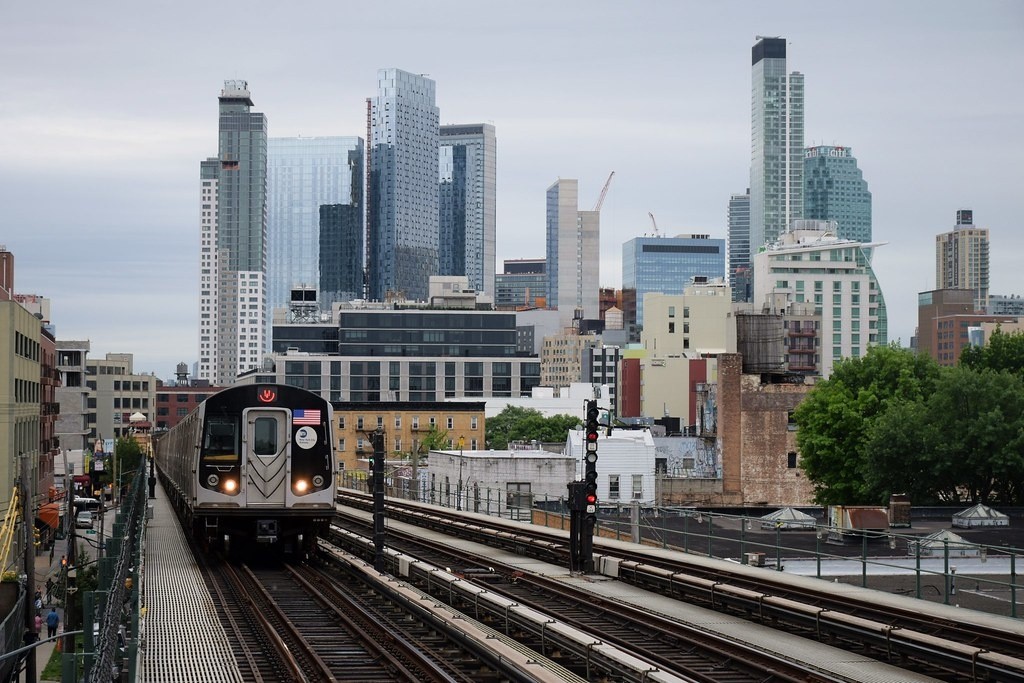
[38,504,59,528]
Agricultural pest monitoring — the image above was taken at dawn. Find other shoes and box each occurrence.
[39,638,41,641]
[46,603,52,605]
[46,638,57,643]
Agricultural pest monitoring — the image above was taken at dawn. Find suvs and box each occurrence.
[76,510,93,529]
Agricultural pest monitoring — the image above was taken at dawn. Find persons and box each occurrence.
[35,613,43,641]
[59,555,66,572]
[35,585,45,618]
[45,578,55,602]
[46,607,60,642]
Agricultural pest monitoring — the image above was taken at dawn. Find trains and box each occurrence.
[155,383,337,556]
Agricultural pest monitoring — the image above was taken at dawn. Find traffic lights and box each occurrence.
[583,398,599,526]
